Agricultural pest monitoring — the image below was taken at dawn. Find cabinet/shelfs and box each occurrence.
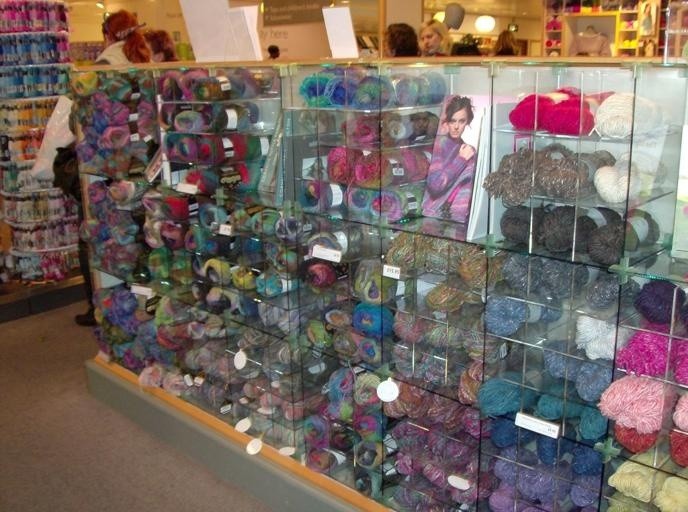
[0,1,688,512]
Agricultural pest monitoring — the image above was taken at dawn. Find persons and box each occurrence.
[381,22,418,57]
[421,94,477,223]
[268,45,280,58]
[418,19,453,56]
[495,29,521,55]
[568,25,612,57]
[53,9,152,326]
[644,39,654,55]
[640,3,653,35]
[442,2,465,30]
[143,30,179,61]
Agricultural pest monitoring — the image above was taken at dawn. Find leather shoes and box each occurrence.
[76,314,95,326]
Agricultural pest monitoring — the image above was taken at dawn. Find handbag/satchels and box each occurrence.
[53,148,81,202]
